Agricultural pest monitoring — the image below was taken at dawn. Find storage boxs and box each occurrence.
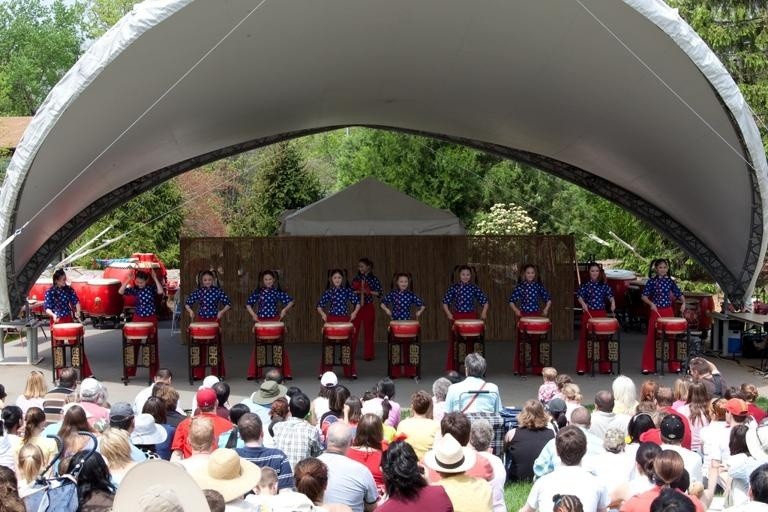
[726,328,741,354]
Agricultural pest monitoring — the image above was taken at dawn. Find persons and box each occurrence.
[316,269,361,379]
[44,269,96,381]
[379,272,427,378]
[173,289,181,321]
[1,352,767,512]
[118,270,163,382]
[641,258,686,375]
[185,270,232,381]
[350,258,383,361]
[508,263,553,376]
[442,265,490,372]
[245,269,295,380]
[575,262,616,375]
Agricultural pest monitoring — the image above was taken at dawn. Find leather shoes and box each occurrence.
[91,367,685,382]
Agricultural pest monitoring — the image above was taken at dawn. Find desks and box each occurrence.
[708,311,767,376]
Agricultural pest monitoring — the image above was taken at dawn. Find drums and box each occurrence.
[671,291,714,332]
[53,323,83,340]
[629,281,651,315]
[323,321,353,340]
[607,278,637,308]
[453,320,484,337]
[519,317,550,336]
[124,321,155,339]
[28,252,162,316]
[587,318,619,335]
[190,321,220,339]
[390,321,421,339]
[656,317,688,335]
[254,322,284,339]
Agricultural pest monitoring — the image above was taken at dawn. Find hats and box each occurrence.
[129,413,168,445]
[603,428,627,454]
[319,370,339,387]
[203,375,219,390]
[196,386,218,407]
[719,397,748,418]
[110,448,262,512]
[424,433,476,473]
[252,380,288,405]
[79,377,102,401]
[109,401,135,423]
[660,414,685,441]
